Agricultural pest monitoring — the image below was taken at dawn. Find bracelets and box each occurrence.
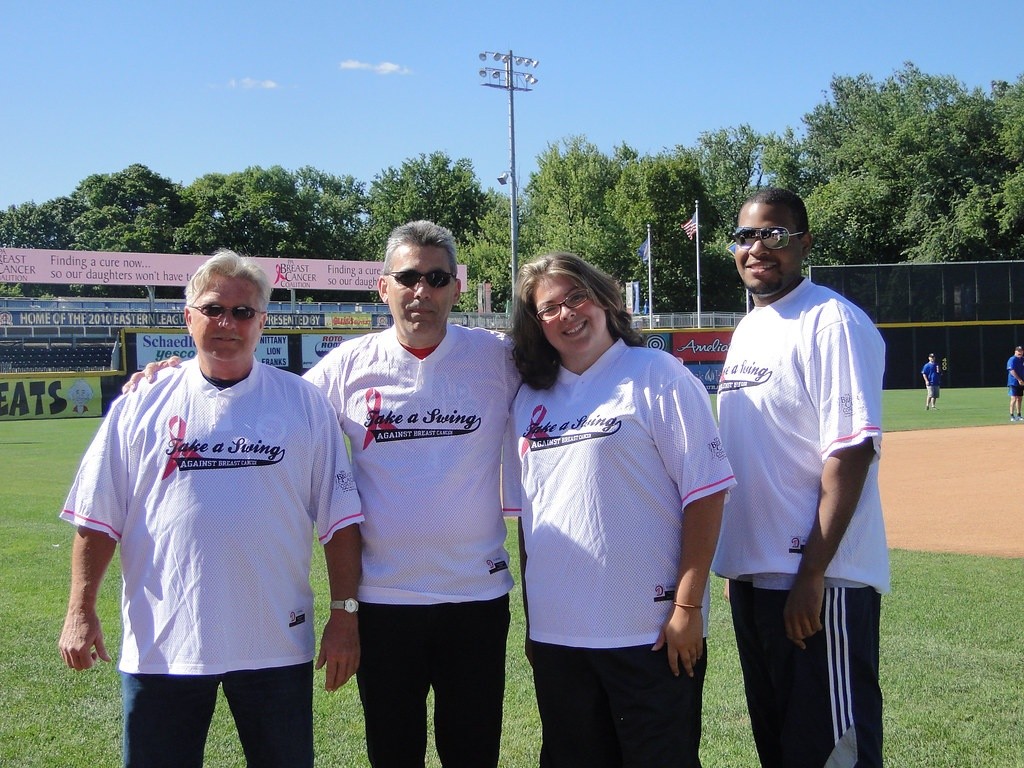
[673,603,702,609]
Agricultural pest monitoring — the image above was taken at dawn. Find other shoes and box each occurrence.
[926,405,930,410]
[1010,416,1015,421]
[932,407,939,410]
[1016,417,1024,421]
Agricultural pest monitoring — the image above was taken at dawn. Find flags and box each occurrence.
[681,211,696,240]
[636,238,648,264]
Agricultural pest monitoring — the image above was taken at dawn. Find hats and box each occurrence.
[1015,346,1022,351]
[929,353,935,357]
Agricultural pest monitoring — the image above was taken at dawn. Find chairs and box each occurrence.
[0,341,114,371]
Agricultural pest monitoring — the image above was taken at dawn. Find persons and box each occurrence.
[712,188,886,768]
[1007,345,1024,421]
[738,233,747,245]
[121,220,683,768]
[501,252,738,768]
[920,352,941,410]
[58,251,364,768]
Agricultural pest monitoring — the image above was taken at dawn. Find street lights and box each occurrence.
[476,49,540,302]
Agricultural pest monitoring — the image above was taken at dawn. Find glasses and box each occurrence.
[385,270,455,287]
[188,304,264,321]
[733,226,803,249]
[536,289,589,322]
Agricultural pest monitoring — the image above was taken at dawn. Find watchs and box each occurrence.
[330,598,360,614]
